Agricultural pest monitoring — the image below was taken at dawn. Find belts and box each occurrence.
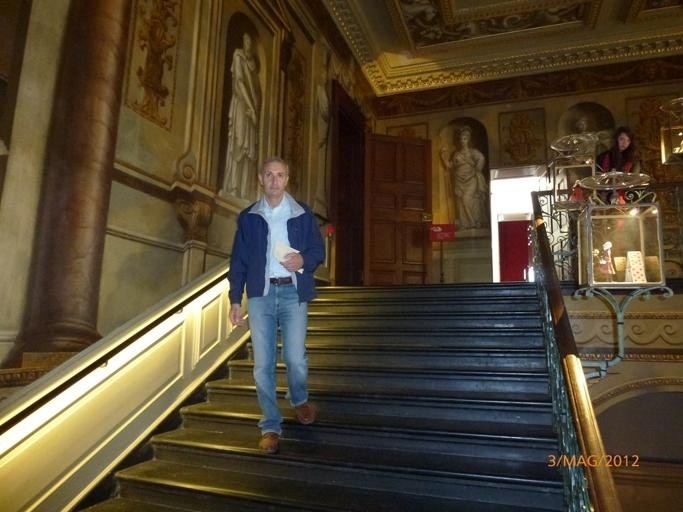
[268,276,292,285]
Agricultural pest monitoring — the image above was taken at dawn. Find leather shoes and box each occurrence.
[256,432,279,454]
[295,401,315,425]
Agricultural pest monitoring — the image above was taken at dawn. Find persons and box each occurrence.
[439,126,487,229]
[594,126,642,256]
[225,32,262,199]
[227,157,327,450]
